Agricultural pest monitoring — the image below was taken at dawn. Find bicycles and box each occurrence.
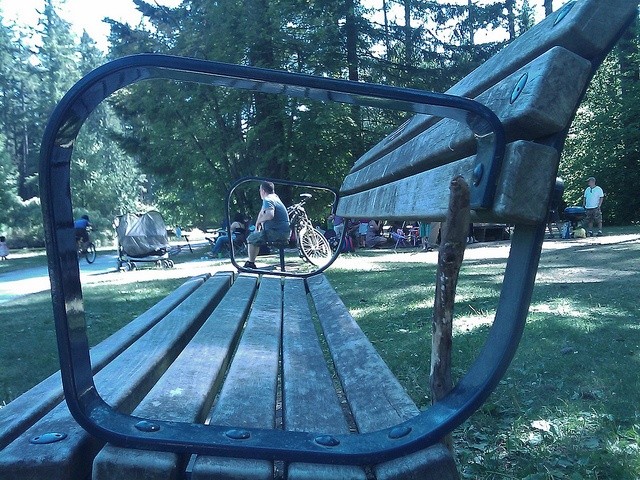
[169,235,193,257]
[79,229,98,264]
[287,194,331,267]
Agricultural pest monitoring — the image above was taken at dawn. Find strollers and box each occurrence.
[113,215,174,272]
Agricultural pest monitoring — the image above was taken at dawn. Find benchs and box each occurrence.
[0,0,640,480]
[261,213,298,271]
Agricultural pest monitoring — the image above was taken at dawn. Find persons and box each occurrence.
[419,221,434,251]
[204,212,246,258]
[73,214,91,249]
[547,194,563,240]
[582,174,605,236]
[329,212,345,240]
[0,235,9,262]
[365,218,388,247]
[174,225,182,239]
[242,181,291,270]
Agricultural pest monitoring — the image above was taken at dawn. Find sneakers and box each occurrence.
[238,261,256,272]
